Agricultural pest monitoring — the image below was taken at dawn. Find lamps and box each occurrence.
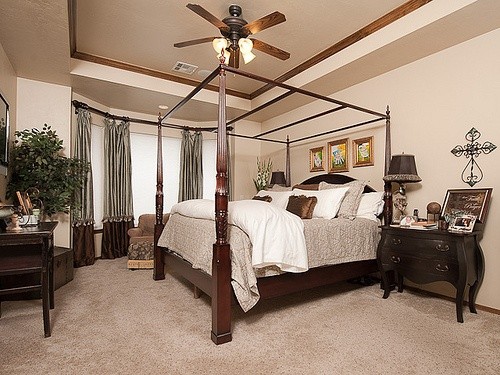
[269,169,288,187]
[383,152,422,225]
[238,38,256,65]
[212,37,231,65]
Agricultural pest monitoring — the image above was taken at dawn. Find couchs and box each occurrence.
[128,214,169,271]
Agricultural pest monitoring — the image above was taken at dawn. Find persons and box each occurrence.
[458,218,468,227]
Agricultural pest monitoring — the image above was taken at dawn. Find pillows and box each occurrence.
[253,184,383,222]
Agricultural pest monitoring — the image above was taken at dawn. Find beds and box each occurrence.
[153,48,394,345]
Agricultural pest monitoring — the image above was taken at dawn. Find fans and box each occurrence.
[173,4,290,60]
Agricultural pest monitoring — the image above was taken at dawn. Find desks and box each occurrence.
[0,221,58,338]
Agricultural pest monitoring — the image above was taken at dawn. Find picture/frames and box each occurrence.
[0,92,10,168]
[327,138,350,173]
[352,136,374,168]
[16,191,34,215]
[451,215,477,233]
[441,188,493,224]
[309,146,326,172]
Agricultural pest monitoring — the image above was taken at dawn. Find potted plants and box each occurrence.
[5,121,92,301]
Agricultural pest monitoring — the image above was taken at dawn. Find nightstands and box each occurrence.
[376,225,485,323]
